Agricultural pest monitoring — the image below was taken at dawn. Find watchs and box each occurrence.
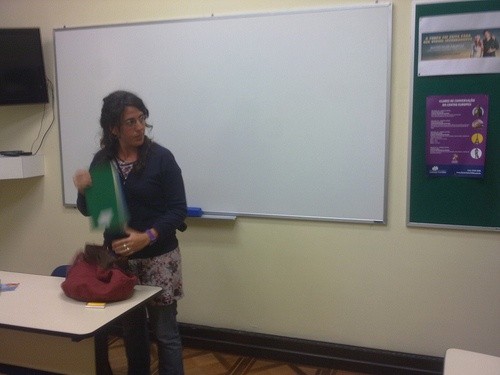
[145,229,158,246]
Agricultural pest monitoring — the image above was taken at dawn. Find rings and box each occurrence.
[127,247,130,251]
[123,244,126,248]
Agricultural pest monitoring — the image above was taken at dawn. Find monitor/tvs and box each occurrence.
[0,28,50,106]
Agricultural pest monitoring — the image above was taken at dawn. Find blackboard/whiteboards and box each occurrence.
[53,2,393,226]
[405,0,500,234]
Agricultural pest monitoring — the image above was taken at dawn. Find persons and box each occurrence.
[72,90,188,375]
[473,30,499,58]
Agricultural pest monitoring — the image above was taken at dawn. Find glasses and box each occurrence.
[119,113,147,128]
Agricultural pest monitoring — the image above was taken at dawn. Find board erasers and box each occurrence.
[186,207,201,217]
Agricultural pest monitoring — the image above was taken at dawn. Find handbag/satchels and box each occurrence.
[60,251,138,304]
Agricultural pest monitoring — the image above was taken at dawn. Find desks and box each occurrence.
[0,270,163,375]
